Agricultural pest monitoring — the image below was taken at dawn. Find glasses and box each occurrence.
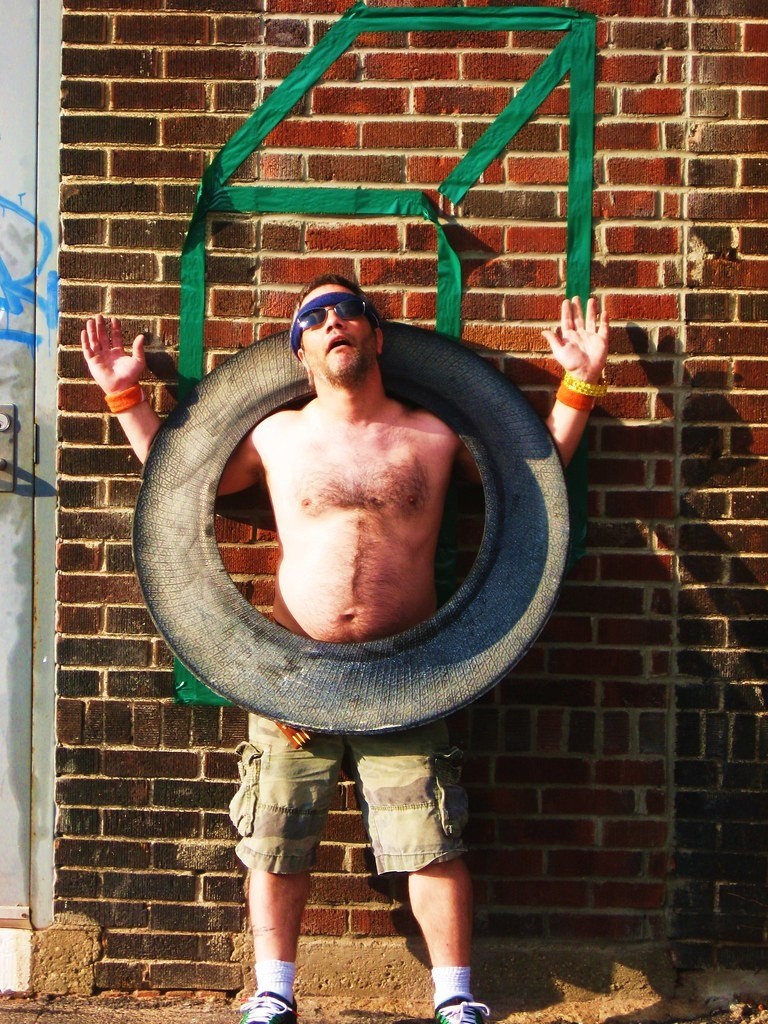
[297,297,380,344]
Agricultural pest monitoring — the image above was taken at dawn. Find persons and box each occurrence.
[81,274,611,1024]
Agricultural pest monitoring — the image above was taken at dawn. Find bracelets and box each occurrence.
[105,383,146,412]
[556,384,596,410]
[563,372,607,398]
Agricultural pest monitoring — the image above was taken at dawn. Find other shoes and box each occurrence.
[239,996,298,1024]
[434,994,492,1024]
